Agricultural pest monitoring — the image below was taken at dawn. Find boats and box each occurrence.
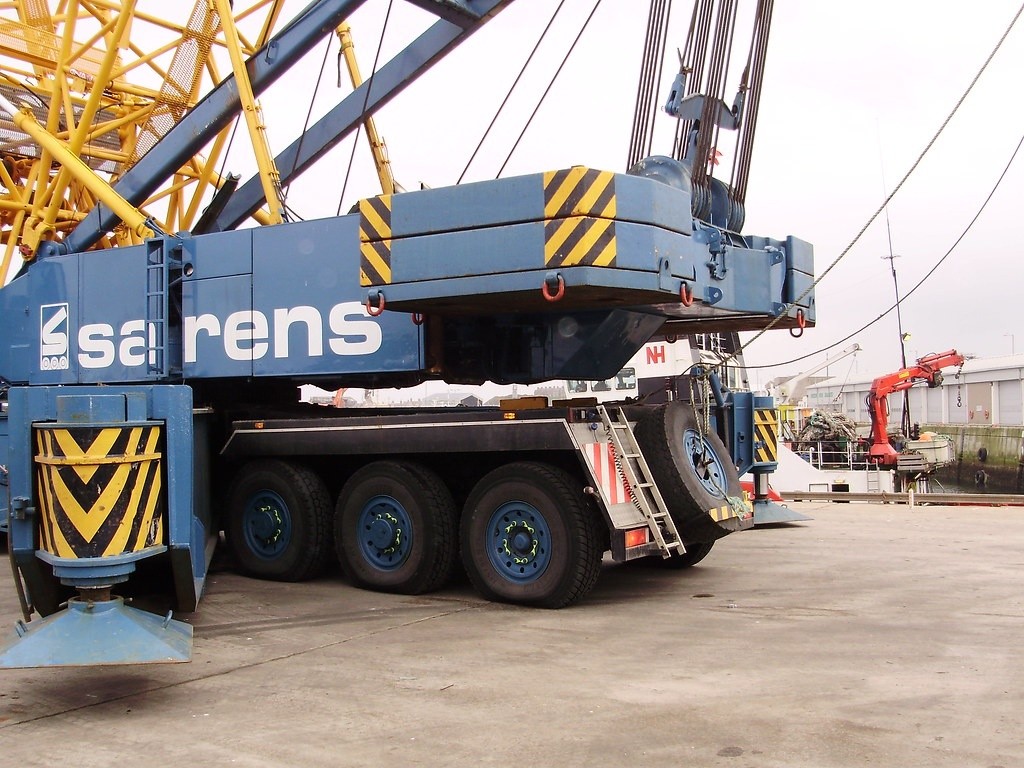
[298,339,978,506]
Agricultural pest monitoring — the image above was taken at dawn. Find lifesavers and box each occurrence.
[974,470,984,485]
[978,448,986,460]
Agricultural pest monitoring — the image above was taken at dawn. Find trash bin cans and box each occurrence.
[831,483,850,503]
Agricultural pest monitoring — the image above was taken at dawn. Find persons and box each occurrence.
[895,429,905,451]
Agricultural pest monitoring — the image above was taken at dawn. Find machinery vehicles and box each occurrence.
[0,0,817,674]
